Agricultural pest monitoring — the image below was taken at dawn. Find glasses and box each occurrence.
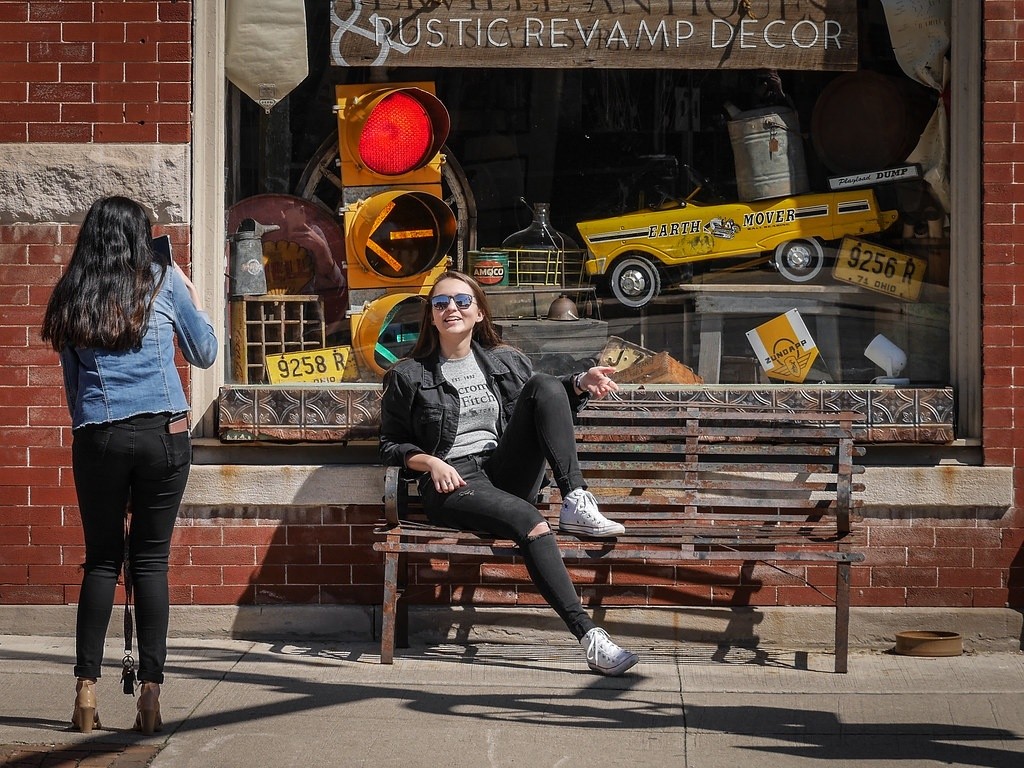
[431,293,479,310]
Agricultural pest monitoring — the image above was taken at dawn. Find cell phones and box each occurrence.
[152,234,173,268]
[286,205,306,243]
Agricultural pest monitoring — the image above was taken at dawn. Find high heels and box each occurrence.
[133,682,165,735]
[71,678,101,734]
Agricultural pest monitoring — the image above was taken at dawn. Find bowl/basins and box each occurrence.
[894,631,963,657]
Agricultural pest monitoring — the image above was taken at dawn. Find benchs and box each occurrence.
[371,406,866,674]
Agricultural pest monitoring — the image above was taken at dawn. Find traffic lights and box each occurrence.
[330,79,462,381]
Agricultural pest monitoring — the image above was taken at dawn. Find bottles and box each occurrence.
[502,203,581,285]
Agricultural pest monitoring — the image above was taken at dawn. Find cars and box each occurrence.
[576,161,897,312]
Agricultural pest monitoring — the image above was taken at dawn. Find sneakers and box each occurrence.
[580,628,639,675]
[558,489,627,538]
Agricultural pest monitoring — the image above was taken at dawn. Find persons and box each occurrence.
[255,195,348,306]
[377,271,639,676]
[40,196,219,737]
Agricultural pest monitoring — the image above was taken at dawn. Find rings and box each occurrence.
[446,482,452,486]
[608,379,612,383]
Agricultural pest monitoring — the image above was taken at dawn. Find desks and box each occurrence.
[680,283,866,383]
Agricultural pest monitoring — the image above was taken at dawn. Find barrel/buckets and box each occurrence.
[726,102,811,203]
[468,251,509,287]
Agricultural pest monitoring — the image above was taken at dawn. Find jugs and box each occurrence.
[229,218,280,296]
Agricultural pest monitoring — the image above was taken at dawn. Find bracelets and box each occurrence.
[575,371,589,392]
